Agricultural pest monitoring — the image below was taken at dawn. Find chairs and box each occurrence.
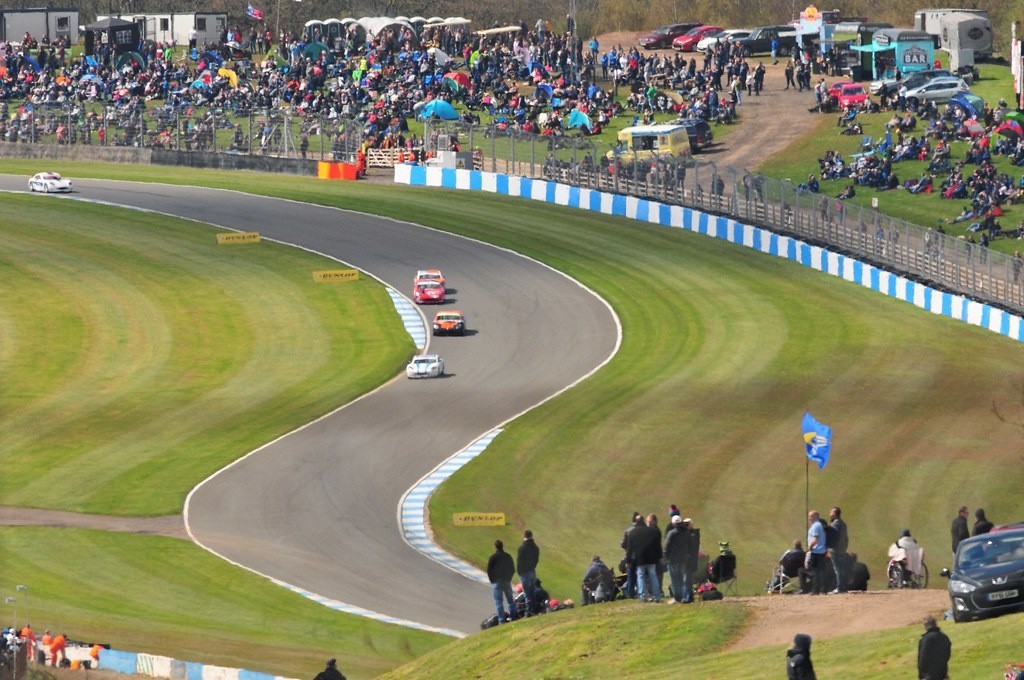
[688,555,739,601]
[582,567,624,605]
[770,549,807,595]
[962,540,1024,568]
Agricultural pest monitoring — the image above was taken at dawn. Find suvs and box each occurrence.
[655,118,713,155]
[730,26,796,58]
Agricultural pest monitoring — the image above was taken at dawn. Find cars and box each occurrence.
[904,79,971,108]
[414,270,446,288]
[829,82,870,113]
[672,25,725,52]
[432,310,466,336]
[869,77,896,96]
[406,354,444,379]
[638,23,704,49]
[414,281,446,304]
[939,529,1024,623]
[983,522,1024,551]
[879,69,953,98]
[696,30,754,56]
[28,172,72,194]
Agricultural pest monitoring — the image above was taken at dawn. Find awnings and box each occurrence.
[850,44,894,51]
[812,36,855,43]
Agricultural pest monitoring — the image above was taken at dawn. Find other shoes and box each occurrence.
[833,588,846,593]
[667,597,679,604]
[799,589,808,594]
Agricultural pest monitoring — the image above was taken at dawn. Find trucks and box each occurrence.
[605,125,691,173]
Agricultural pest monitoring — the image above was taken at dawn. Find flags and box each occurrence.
[802,412,831,468]
[246,2,265,20]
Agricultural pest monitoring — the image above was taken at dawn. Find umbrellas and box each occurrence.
[226,41,244,50]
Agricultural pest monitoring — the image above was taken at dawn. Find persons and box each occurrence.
[313,658,346,680]
[580,504,732,606]
[783,43,1024,284]
[951,506,994,555]
[786,633,816,680]
[917,616,951,680]
[765,507,871,595]
[0,15,780,206]
[0,624,68,667]
[886,528,917,586]
[487,530,549,625]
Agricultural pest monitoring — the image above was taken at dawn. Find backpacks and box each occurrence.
[819,521,836,548]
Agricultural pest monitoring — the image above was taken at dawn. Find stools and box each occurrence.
[518,599,536,618]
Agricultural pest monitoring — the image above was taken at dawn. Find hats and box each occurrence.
[901,529,909,536]
[719,541,730,551]
[327,658,336,666]
[535,579,542,583]
[668,504,679,517]
[975,508,985,519]
[671,515,682,524]
[516,584,522,592]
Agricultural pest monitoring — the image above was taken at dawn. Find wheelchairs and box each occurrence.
[886,544,929,588]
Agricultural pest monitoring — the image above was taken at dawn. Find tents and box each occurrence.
[303,41,337,64]
[428,47,448,65]
[116,52,146,72]
[421,99,459,121]
[80,18,139,54]
[441,72,472,93]
